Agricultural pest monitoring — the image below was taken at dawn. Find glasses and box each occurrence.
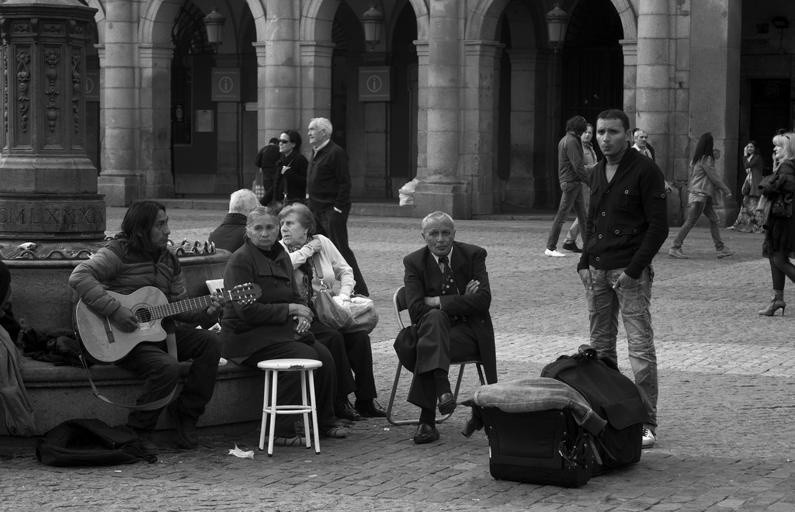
[278,139,288,143]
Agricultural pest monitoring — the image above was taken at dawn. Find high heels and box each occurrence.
[759,289,786,317]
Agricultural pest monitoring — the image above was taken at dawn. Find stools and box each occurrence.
[258,359,324,456]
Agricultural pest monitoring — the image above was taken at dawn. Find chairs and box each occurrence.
[385,284,488,424]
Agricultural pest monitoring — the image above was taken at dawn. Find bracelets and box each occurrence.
[303,245,315,253]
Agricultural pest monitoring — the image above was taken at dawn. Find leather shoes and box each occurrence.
[168,392,457,449]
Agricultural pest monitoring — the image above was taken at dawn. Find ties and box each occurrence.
[441,256,463,325]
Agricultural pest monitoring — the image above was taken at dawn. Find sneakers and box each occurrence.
[544,249,565,257]
[669,248,689,259]
[641,428,655,448]
[717,245,734,258]
[562,241,583,252]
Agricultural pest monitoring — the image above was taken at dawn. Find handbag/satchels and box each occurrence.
[484,407,594,487]
[33,329,93,368]
[313,282,378,334]
[545,344,648,476]
[36,418,159,466]
[267,200,286,214]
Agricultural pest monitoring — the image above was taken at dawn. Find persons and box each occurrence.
[214,206,351,446]
[543,115,602,258]
[668,132,735,259]
[573,107,668,447]
[391,208,496,444]
[66,200,227,455]
[726,141,766,233]
[758,131,795,317]
[274,200,388,418]
[631,127,655,162]
[1,258,40,438]
[254,114,369,300]
[208,189,264,254]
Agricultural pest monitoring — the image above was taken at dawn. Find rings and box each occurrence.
[303,319,306,325]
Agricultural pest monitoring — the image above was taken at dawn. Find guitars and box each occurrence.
[74,282,263,363]
